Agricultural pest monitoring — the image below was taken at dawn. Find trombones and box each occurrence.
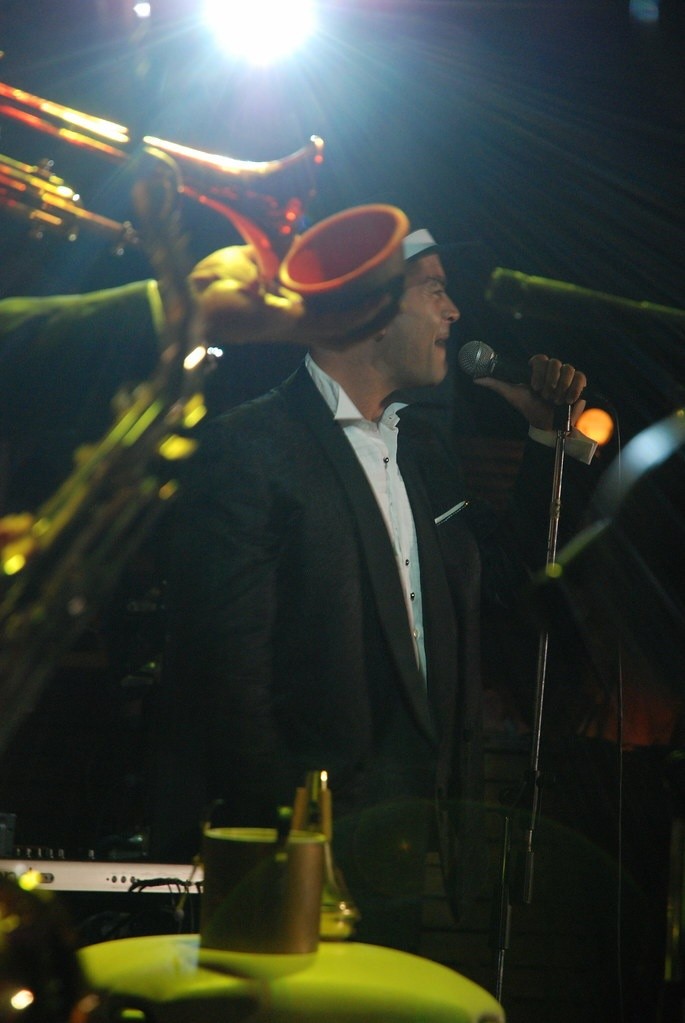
[0,78,325,290]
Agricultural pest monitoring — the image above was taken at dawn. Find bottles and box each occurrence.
[290,769,361,943]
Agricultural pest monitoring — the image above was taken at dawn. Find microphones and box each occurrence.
[458,340,610,407]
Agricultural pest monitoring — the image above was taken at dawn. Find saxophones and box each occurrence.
[1,142,230,765]
[0,152,164,259]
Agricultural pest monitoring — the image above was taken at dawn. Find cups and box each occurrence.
[196,828,327,976]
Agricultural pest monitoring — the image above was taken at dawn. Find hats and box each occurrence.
[298,179,437,261]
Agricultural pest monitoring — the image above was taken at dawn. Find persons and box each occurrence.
[0,243,387,386]
[184,196,590,999]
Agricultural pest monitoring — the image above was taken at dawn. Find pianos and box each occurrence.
[1,840,208,899]
